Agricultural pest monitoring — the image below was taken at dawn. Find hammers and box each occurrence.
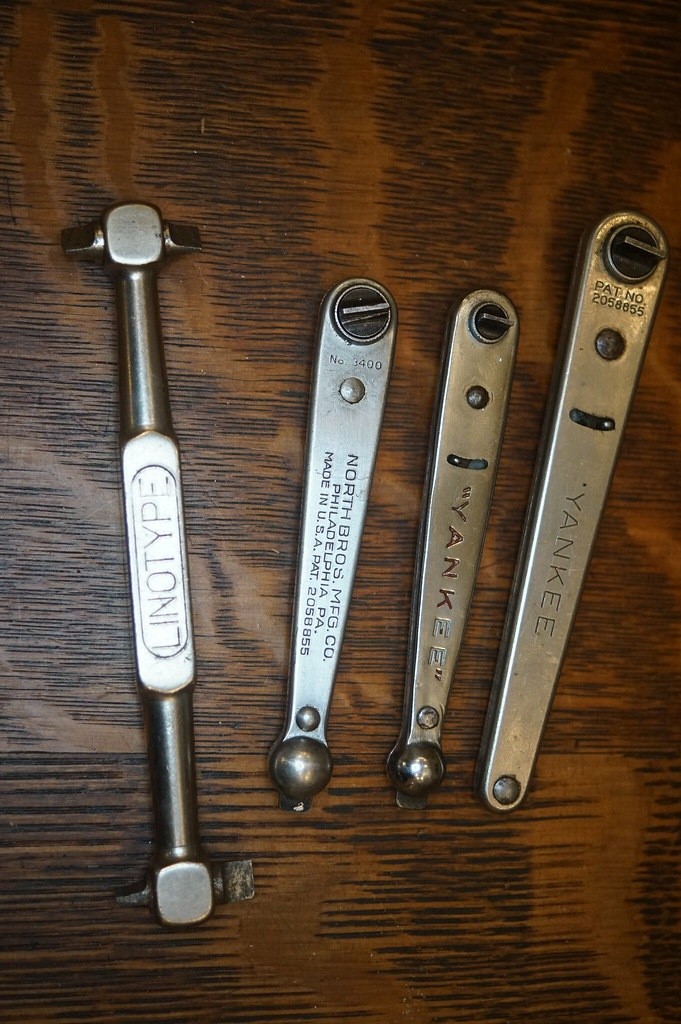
[55,196,258,925]
